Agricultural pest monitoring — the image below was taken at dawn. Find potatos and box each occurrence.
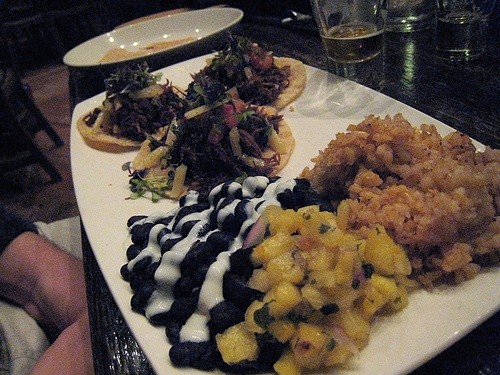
[215,199,413,375]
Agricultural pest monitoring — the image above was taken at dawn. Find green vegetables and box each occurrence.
[106,32,272,202]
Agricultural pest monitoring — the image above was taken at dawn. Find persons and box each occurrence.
[0,207,97,375]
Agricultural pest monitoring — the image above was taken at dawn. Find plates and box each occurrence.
[63,8,243,71]
[70,47,500,375]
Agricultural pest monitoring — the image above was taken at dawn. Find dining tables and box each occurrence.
[68,0,500,375]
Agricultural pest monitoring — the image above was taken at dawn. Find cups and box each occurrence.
[436,0,496,24]
[386,0,438,31]
[309,0,388,64]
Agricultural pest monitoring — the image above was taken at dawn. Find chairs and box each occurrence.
[0,58,63,184]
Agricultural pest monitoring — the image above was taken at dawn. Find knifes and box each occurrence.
[245,13,317,31]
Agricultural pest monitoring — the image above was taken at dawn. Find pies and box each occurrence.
[265,57,306,112]
[132,106,296,195]
[77,107,143,146]
